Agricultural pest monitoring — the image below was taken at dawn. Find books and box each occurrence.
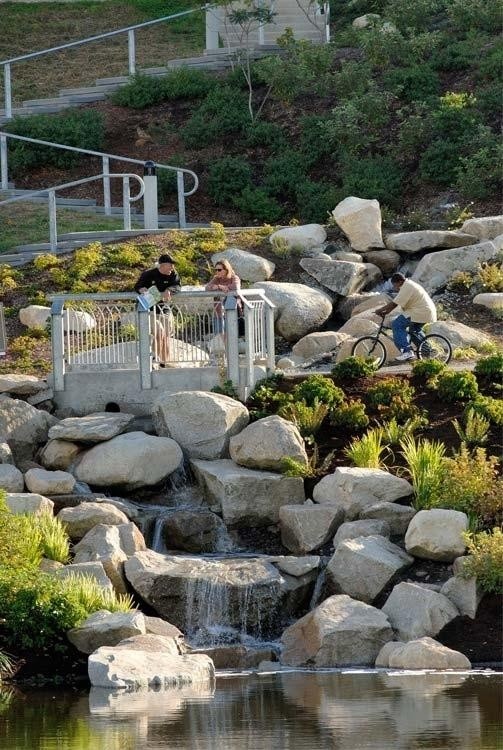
[137,285,162,311]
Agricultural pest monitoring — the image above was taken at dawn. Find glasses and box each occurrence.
[214,267,223,272]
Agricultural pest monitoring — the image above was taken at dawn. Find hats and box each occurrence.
[158,252,180,265]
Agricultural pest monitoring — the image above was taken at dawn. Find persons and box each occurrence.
[134,254,182,367]
[375,273,437,362]
[205,259,242,357]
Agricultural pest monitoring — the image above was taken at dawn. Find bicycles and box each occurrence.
[351,312,452,377]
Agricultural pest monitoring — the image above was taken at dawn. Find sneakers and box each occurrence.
[394,350,417,361]
